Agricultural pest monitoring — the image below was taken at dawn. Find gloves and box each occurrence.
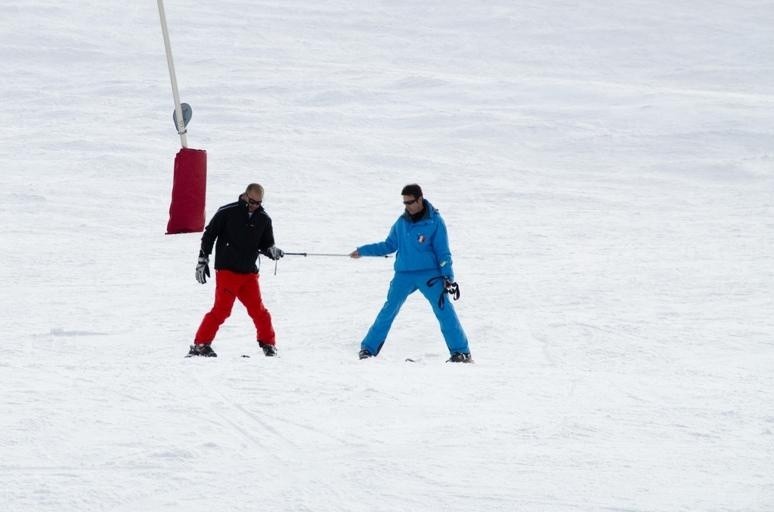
[444,280,459,294]
[268,247,282,261]
[195,252,211,284]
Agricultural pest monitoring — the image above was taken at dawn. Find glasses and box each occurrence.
[403,199,418,205]
[247,195,261,206]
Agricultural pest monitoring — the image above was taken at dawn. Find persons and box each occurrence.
[189,183,283,357]
[351,184,472,363]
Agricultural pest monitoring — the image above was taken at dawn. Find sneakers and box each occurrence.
[189,345,216,357]
[449,353,471,363]
[359,349,371,359]
[264,344,276,354]
